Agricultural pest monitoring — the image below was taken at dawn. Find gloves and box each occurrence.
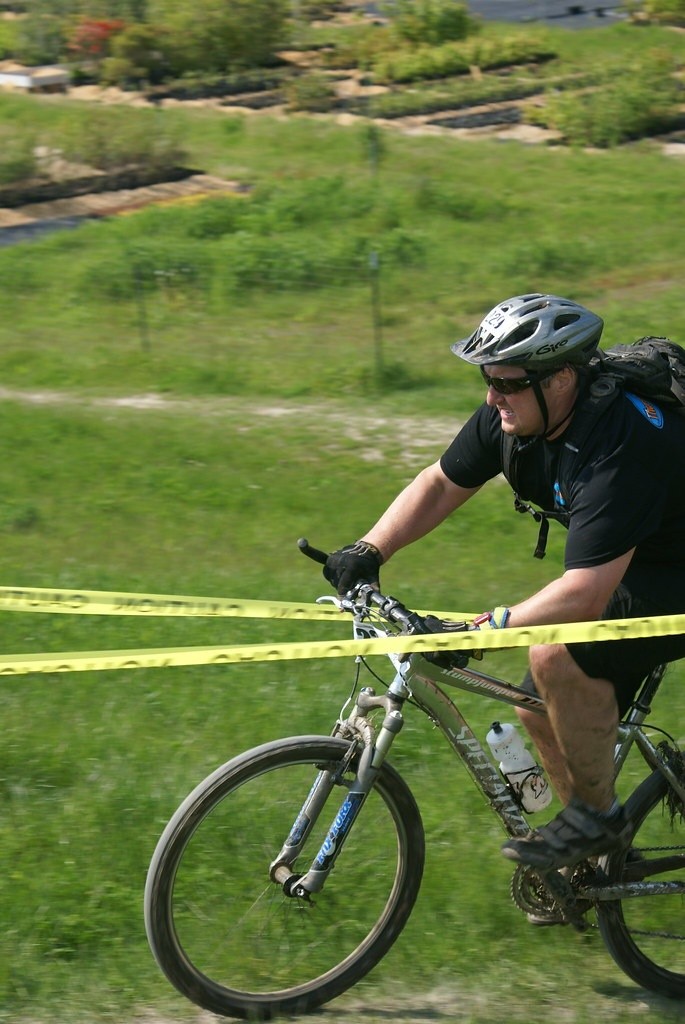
[323,540,384,599]
[424,615,473,671]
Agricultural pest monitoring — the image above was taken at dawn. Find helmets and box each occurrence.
[450,293,604,369]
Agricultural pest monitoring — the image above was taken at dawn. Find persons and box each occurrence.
[322,293,684,926]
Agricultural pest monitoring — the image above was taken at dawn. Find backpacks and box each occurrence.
[503,336,685,559]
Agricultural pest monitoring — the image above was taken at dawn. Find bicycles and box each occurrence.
[145,539,685,1020]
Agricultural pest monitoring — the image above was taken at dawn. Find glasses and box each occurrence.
[480,364,563,395]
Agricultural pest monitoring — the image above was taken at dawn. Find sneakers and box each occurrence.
[502,796,628,870]
[527,846,645,926]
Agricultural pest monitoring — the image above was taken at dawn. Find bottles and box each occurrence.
[486,721,553,814]
